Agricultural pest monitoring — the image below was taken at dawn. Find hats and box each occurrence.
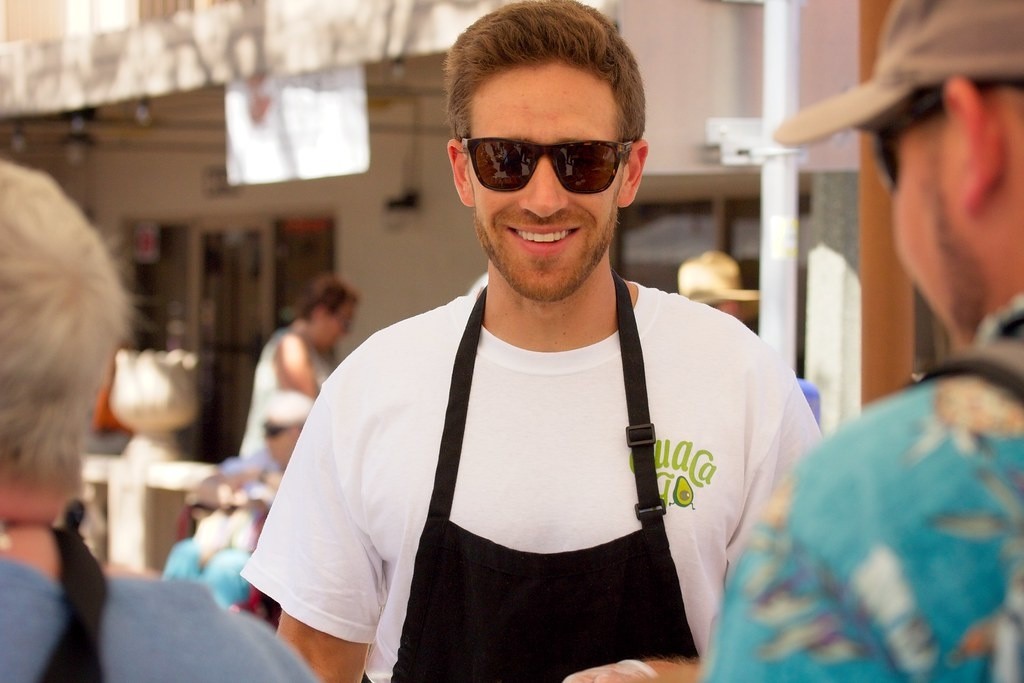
[678,252,761,321]
[776,0,1023,150]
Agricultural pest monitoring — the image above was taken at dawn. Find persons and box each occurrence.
[2,164,326,683]
[155,269,360,637]
[667,249,762,333]
[240,0,822,683]
[700,0,1024,683]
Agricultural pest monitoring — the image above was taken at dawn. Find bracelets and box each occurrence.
[619,658,658,680]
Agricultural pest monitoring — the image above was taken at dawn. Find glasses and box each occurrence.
[461,137,633,194]
